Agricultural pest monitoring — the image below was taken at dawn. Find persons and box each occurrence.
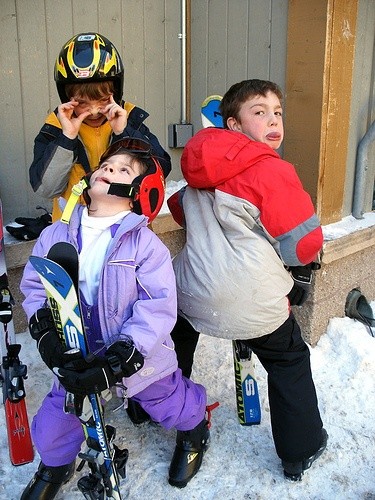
[21,137,213,500]
[168,79,329,480]
[30,32,172,232]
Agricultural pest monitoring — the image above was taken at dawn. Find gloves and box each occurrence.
[282,259,321,307]
[5,212,53,242]
[29,308,72,372]
[52,342,144,394]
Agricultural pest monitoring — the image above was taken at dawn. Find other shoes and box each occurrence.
[281,427,328,482]
[19,460,74,500]
[166,419,211,488]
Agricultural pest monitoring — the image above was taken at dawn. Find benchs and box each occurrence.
[4,213,186,334]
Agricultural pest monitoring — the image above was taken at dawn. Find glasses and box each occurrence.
[98,137,153,157]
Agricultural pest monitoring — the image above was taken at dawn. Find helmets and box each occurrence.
[54,32,124,106]
[80,136,166,225]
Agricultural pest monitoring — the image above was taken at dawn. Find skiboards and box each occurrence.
[28,241,127,499]
[201,96,261,425]
[0,195,36,466]
